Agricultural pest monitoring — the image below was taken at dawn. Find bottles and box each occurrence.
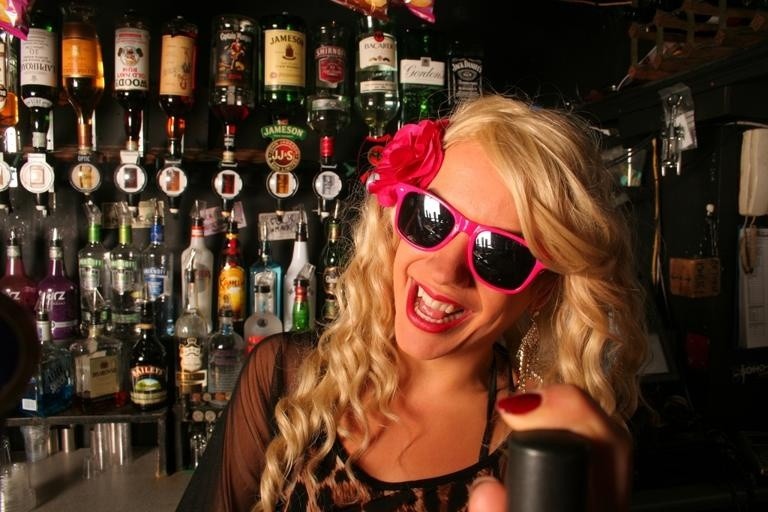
[320,219,350,324]
[400,13,447,121]
[167,271,211,425]
[248,220,284,319]
[73,309,125,413]
[448,20,485,106]
[61,19,106,152]
[216,219,249,327]
[79,215,108,310]
[160,15,199,159]
[106,213,141,311]
[244,281,282,358]
[23,311,75,413]
[280,222,318,331]
[125,300,168,415]
[113,16,153,152]
[211,312,245,416]
[178,218,216,333]
[290,278,309,332]
[310,16,351,164]
[34,229,77,341]
[258,14,309,141]
[17,20,55,152]
[133,216,176,335]
[354,10,398,137]
[1,229,37,309]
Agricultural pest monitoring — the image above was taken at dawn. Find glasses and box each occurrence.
[391,182,550,296]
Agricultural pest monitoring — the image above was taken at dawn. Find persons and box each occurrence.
[174,95,654,512]
